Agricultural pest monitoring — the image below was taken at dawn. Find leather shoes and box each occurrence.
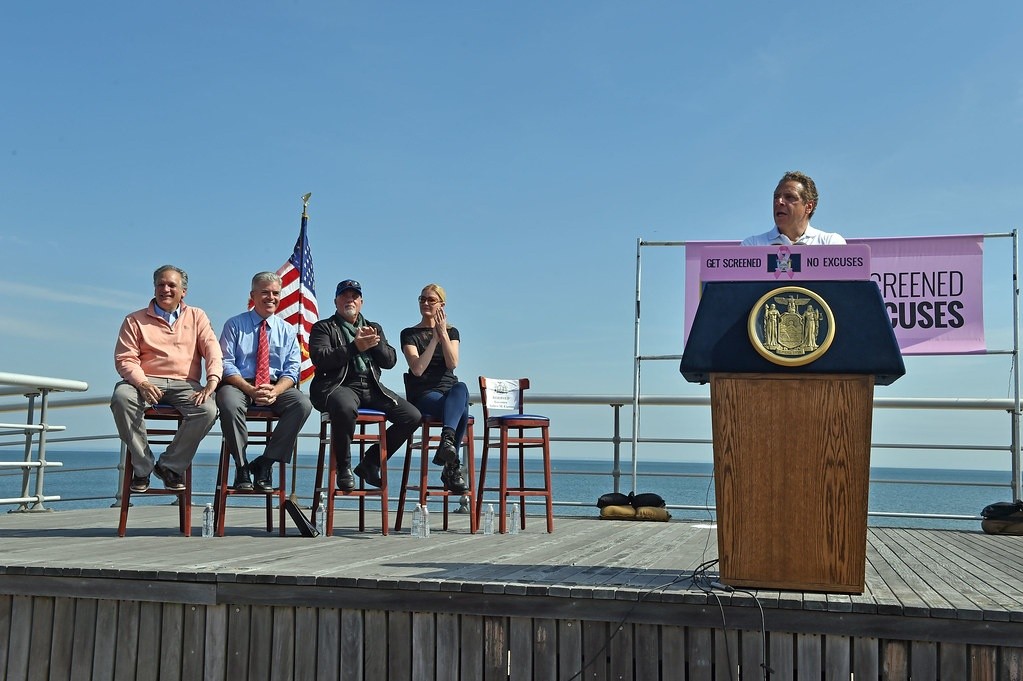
[353,458,381,488]
[337,469,356,492]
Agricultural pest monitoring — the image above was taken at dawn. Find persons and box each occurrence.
[109,266,223,492]
[213,271,312,492]
[738,171,847,246]
[308,279,422,491]
[400,283,470,493]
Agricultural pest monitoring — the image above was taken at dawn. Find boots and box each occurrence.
[440,460,469,494]
[432,429,456,467]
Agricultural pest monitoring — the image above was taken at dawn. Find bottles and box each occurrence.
[411,504,429,537]
[508,503,520,533]
[316,502,326,536]
[484,503,494,533]
[201,503,214,536]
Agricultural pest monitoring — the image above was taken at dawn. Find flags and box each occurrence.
[248,217,320,384]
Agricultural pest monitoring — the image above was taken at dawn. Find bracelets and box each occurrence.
[207,374,220,381]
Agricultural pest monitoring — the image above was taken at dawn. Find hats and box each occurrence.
[336,279,362,297]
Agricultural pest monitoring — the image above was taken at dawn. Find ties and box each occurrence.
[255,320,271,388]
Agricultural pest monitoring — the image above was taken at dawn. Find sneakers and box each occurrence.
[233,466,253,491]
[130,473,150,492]
[152,461,186,490]
[249,460,273,492]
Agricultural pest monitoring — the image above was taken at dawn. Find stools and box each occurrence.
[395,373,478,534]
[118,402,192,537]
[311,406,389,535]
[478,376,553,533]
[214,404,287,537]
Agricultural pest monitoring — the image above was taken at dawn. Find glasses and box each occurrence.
[417,296,443,304]
[337,280,361,290]
